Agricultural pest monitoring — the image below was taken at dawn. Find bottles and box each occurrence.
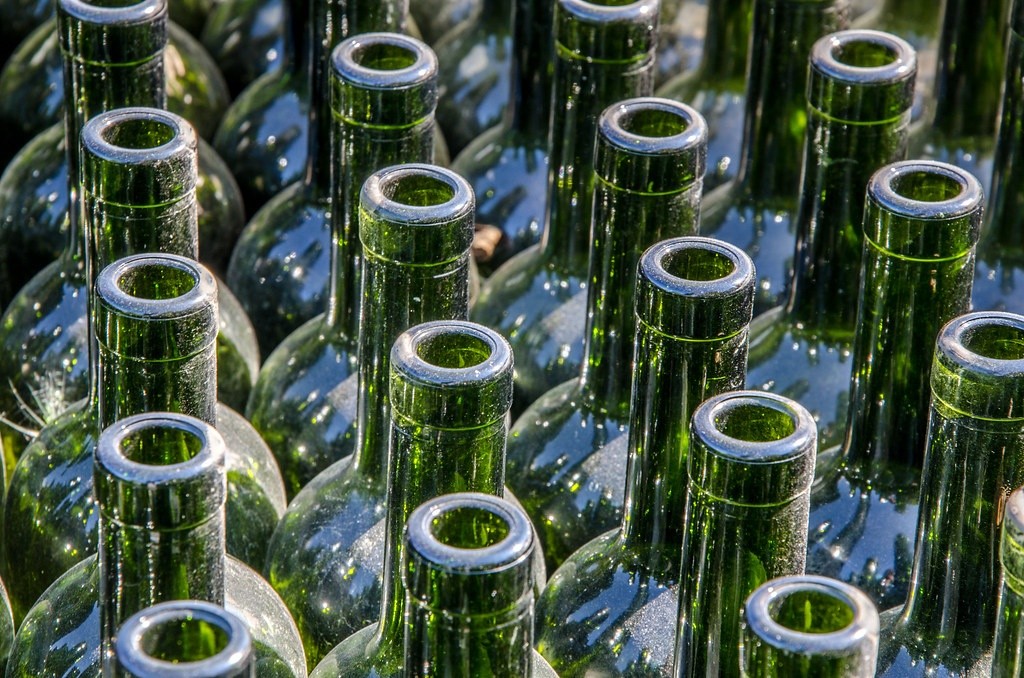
[0,0,1024,678]
